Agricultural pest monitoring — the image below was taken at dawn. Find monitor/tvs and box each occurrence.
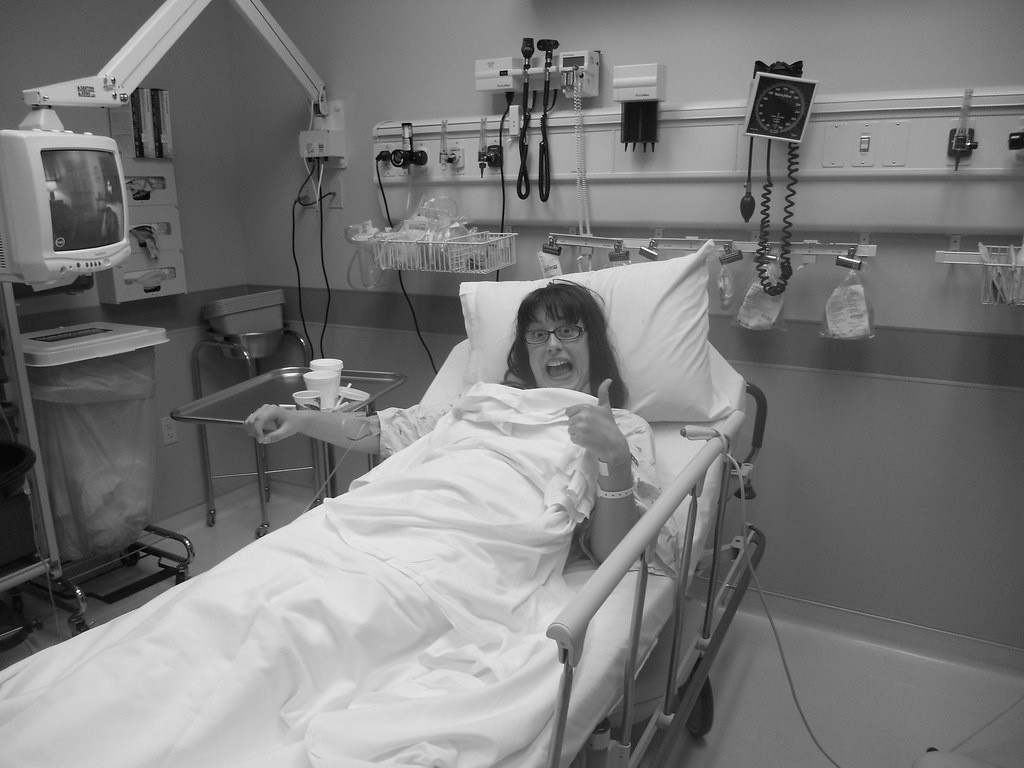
[0,128,130,284]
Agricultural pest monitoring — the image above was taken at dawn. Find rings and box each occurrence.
[251,413,254,416]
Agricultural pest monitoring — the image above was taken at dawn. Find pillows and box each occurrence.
[451,238,747,425]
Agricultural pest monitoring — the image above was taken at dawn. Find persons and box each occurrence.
[242,278,680,565]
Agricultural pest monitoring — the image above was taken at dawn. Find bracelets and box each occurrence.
[598,453,638,477]
[595,481,635,499]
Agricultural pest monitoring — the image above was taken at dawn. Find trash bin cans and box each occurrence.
[17,321,172,562]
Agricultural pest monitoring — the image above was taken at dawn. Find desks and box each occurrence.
[168,369,406,538]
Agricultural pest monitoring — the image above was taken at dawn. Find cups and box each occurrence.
[292,358,344,412]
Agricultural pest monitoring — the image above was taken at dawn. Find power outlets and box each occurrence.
[447,145,463,171]
[379,143,397,177]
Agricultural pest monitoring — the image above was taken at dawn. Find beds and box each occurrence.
[0,373,770,768]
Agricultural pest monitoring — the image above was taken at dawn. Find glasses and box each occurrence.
[525,325,587,344]
